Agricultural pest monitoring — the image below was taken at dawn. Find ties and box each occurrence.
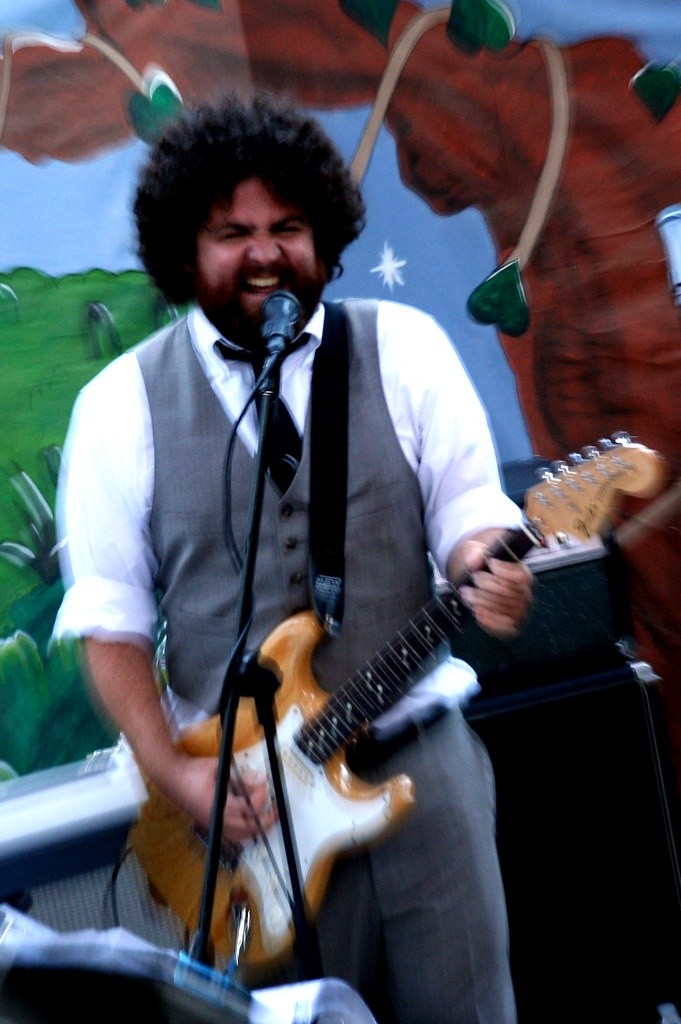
[215,332,309,495]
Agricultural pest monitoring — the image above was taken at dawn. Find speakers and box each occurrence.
[463,665,681,1024]
[0,738,253,998]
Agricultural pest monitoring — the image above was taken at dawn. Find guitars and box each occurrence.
[132,431,662,973]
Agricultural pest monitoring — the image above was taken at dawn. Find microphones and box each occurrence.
[260,290,304,355]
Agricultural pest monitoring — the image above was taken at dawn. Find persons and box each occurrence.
[52,93,533,1024]
